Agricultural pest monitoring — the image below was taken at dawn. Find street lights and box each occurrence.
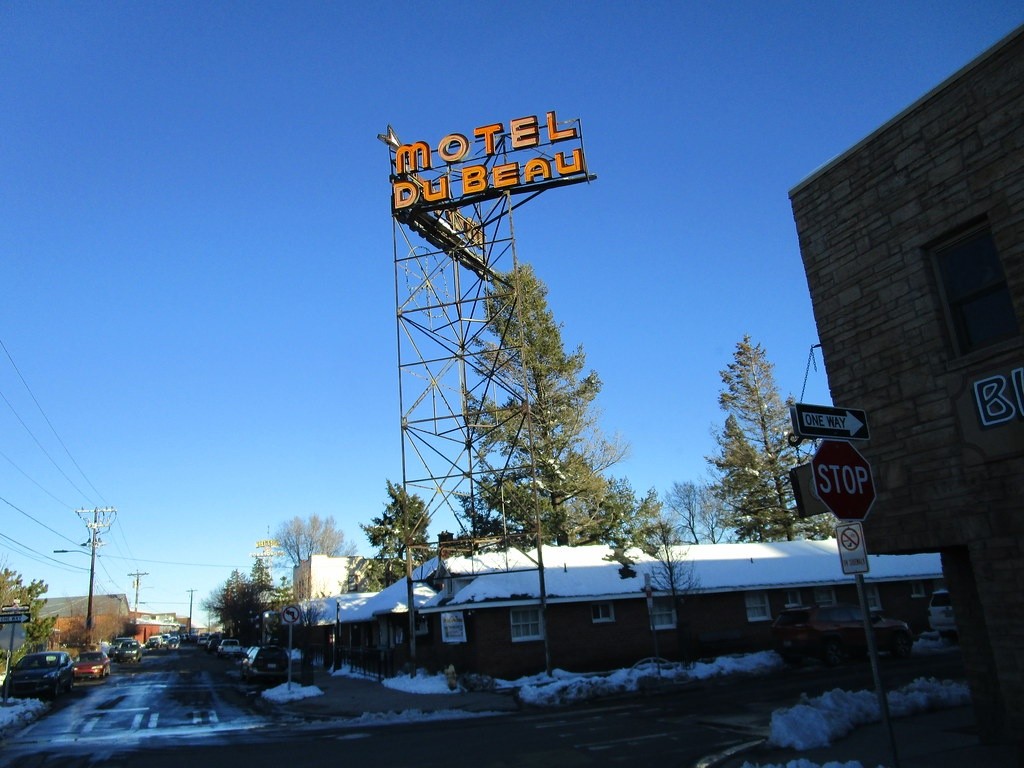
[53,550,95,629]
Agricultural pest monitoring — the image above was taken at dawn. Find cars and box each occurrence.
[926,589,959,641]
[208,640,221,650]
[166,637,180,650]
[113,641,142,664]
[172,631,209,642]
[197,637,208,646]
[4,652,75,699]
[73,651,112,678]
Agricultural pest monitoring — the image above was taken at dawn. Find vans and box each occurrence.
[162,633,170,646]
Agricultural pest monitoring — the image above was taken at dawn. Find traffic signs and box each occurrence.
[794,403,870,440]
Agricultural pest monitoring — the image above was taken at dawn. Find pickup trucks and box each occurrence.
[218,639,242,658]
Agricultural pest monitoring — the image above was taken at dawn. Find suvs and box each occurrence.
[241,647,289,683]
[769,602,915,668]
[145,636,163,649]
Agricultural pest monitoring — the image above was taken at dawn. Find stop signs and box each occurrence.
[810,438,878,523]
